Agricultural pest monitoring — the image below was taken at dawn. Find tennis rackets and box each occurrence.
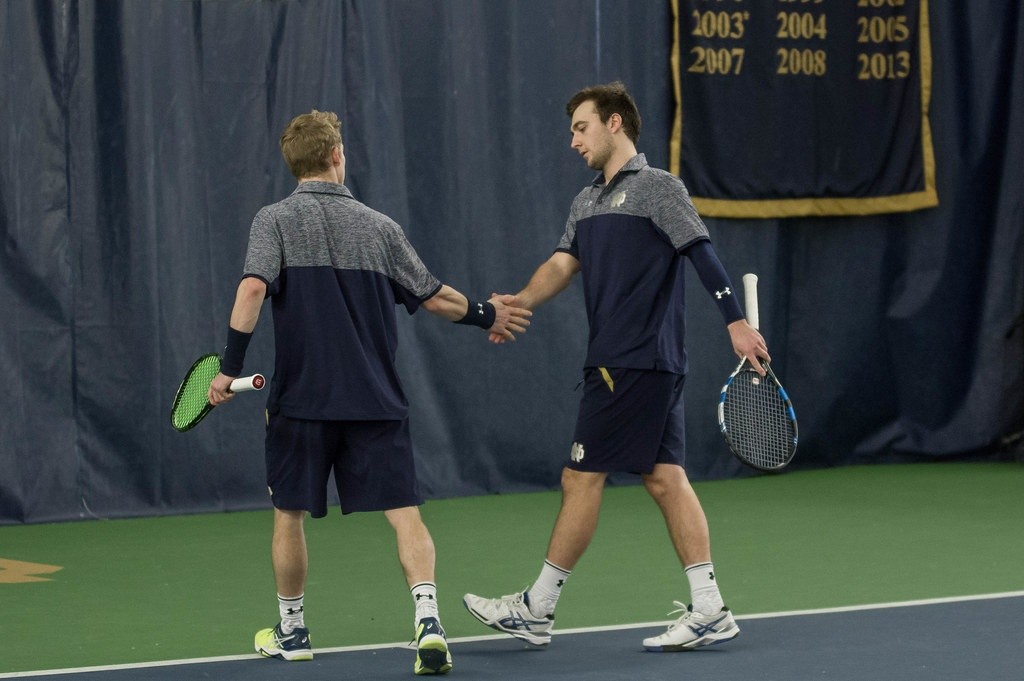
[714,273,800,473]
[170,353,267,433]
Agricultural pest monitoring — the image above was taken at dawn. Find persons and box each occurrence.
[461,84,771,653]
[208,112,533,674]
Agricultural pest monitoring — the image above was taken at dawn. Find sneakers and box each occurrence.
[255,621,313,661]
[642,600,740,652]
[462,586,555,645]
[408,617,452,674]
